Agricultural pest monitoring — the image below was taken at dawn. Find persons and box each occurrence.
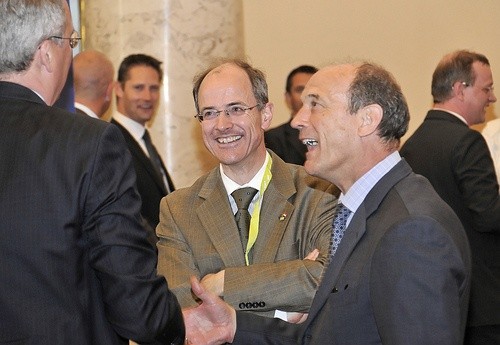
[153,60,342,325]
[0,0,189,345]
[400,47,500,345]
[178,60,471,344]
[262,64,322,168]
[111,53,176,239]
[71,49,114,121]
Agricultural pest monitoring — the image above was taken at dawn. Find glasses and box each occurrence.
[37,26,81,48]
[453,81,497,97]
[193,101,260,122]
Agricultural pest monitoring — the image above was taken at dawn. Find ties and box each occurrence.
[142,128,167,191]
[325,201,352,284]
[230,188,258,261]
